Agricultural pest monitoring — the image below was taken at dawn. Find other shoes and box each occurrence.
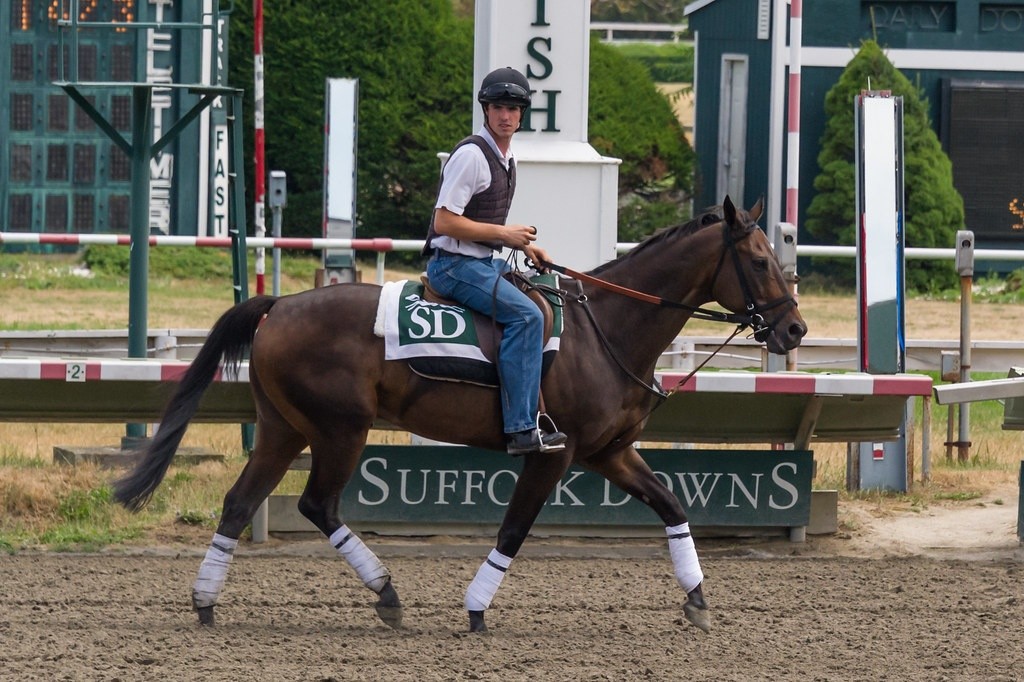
[506,429,568,455]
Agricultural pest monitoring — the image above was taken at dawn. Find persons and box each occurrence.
[419,67,567,457]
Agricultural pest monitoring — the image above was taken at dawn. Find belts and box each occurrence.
[432,249,454,256]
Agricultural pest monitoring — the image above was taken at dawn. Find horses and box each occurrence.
[107,196,808,637]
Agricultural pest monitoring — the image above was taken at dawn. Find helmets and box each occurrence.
[477,66,532,106]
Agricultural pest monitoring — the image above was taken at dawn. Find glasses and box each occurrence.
[478,82,531,105]
